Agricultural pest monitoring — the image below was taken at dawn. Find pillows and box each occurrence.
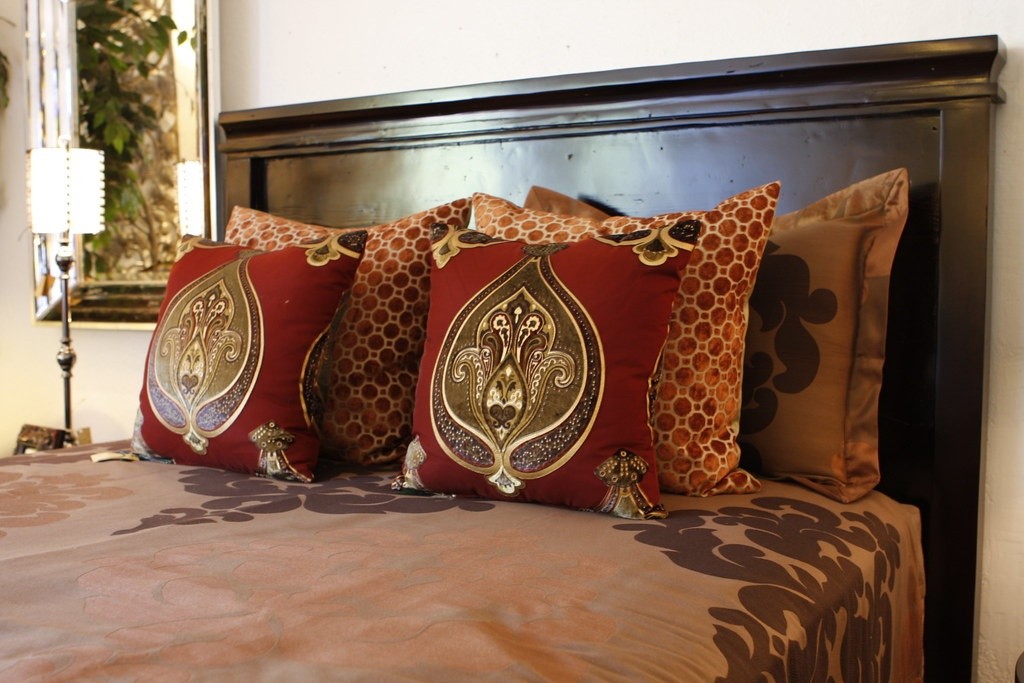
[93,229,369,484]
[526,167,908,504]
[472,180,782,498]
[391,220,702,520]
[223,195,473,473]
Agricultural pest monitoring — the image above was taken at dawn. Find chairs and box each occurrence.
[13,423,63,456]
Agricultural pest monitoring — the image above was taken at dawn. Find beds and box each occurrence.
[0,32,1010,683]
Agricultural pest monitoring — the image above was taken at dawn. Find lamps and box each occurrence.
[26,137,106,447]
